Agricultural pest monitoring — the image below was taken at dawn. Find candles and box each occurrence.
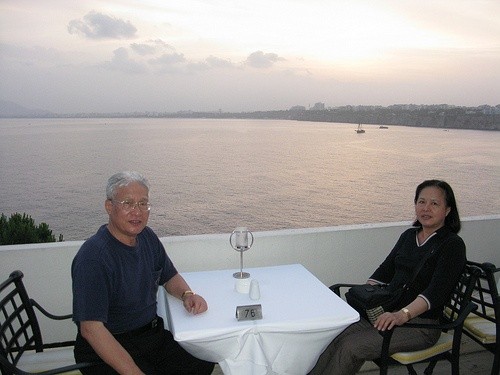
[235,227,248,248]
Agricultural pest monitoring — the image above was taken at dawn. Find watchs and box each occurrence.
[401,308,412,320]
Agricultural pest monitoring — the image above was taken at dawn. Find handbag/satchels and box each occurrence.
[345,282,411,322]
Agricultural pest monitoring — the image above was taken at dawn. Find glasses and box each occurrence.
[108,197,152,212]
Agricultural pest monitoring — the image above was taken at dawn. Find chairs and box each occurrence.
[0,270,95,375]
[329,260,500,375]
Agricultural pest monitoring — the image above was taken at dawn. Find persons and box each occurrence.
[307,180,466,375]
[71,171,214,375]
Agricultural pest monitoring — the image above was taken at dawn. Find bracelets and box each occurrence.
[182,291,193,298]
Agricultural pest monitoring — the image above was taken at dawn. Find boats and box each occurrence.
[379,125,388,129]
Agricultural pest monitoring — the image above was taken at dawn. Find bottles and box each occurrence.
[249,279,260,299]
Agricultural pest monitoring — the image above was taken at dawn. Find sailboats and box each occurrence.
[355,120,365,133]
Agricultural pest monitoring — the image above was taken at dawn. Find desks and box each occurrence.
[164,264,360,375]
